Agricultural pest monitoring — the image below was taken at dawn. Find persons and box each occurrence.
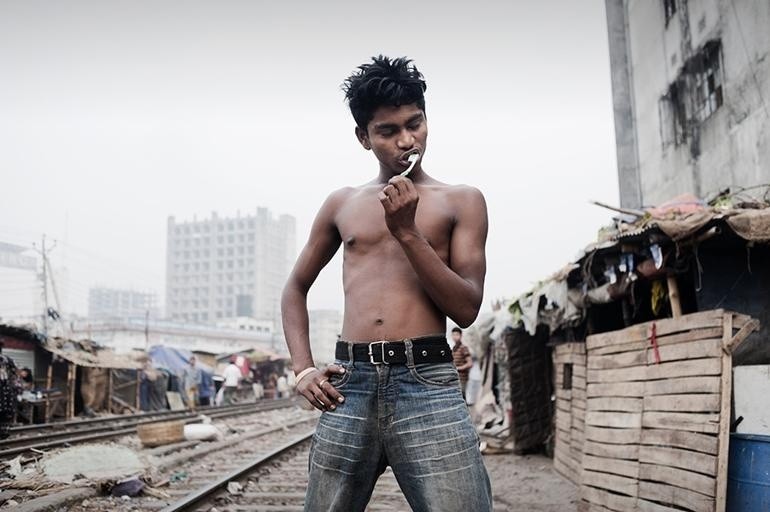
[17,366,34,387]
[138,358,168,412]
[181,355,202,418]
[221,360,242,407]
[478,335,504,430]
[252,378,265,401]
[279,50,496,512]
[266,372,278,391]
[276,373,290,400]
[0,338,17,441]
[451,326,473,408]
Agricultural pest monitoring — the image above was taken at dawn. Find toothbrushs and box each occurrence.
[399,153,420,177]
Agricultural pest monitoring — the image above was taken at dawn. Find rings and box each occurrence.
[319,379,327,388]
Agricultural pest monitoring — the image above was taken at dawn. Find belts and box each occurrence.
[336,338,453,365]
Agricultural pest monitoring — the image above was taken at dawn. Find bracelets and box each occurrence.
[292,366,316,385]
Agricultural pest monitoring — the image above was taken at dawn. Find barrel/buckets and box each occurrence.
[728,433,770,512]
